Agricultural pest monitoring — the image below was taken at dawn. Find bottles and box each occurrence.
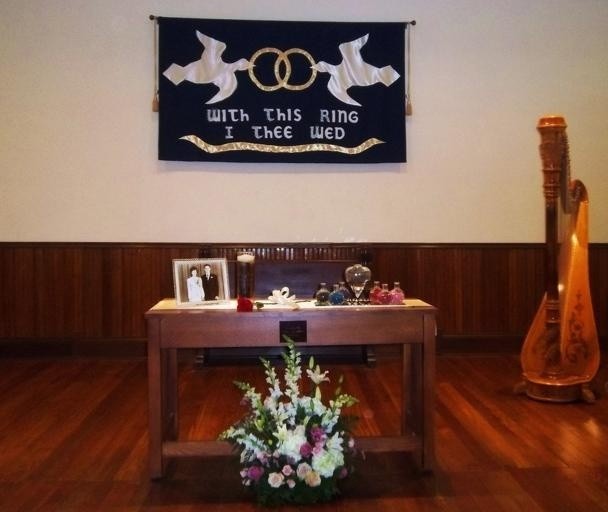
[379,284,392,304]
[338,282,351,300]
[370,281,381,305]
[330,284,343,305]
[390,282,404,304]
[316,283,329,305]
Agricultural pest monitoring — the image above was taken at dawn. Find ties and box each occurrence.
[207,275,209,281]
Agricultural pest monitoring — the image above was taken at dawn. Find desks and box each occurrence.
[146,297,436,479]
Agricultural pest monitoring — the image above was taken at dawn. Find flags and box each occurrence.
[148,14,416,164]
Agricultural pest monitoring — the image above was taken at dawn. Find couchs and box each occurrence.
[198,247,374,365]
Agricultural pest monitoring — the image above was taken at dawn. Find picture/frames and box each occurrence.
[172,257,230,306]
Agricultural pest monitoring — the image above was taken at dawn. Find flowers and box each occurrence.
[217,334,369,505]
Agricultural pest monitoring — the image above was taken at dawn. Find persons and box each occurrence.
[201,264,219,301]
[186,267,205,302]
